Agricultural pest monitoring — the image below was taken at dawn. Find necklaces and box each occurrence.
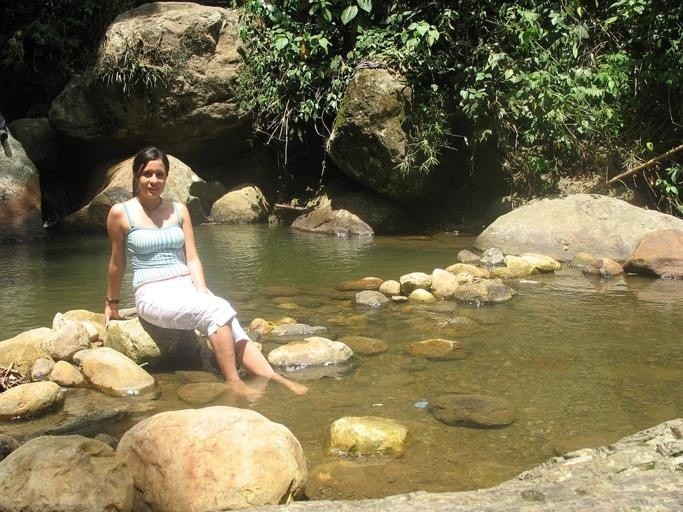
[137,195,163,213]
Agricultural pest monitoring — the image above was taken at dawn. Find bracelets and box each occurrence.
[106,297,120,304]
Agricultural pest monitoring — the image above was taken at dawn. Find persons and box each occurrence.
[104,146,310,398]
